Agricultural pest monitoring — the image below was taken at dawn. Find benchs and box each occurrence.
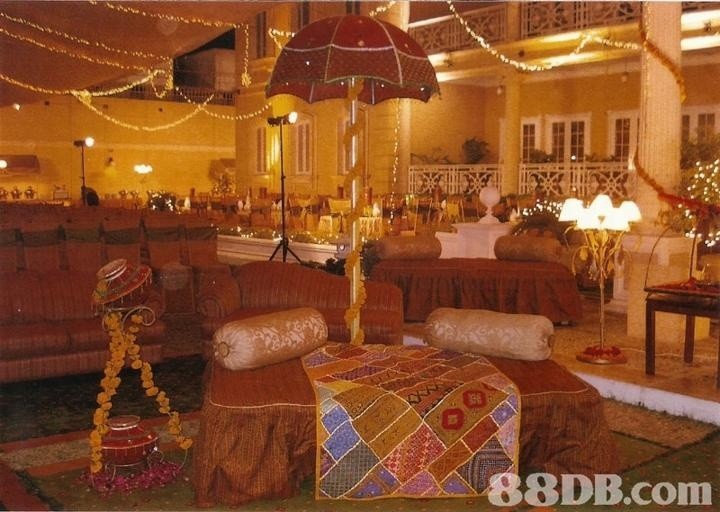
[201,342,600,483]
[373,257,572,326]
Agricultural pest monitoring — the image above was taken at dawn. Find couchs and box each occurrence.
[195,257,406,362]
[0,264,168,385]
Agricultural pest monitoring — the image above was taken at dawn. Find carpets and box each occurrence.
[0,394,719,511]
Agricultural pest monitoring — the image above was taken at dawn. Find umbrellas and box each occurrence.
[263,13,444,351]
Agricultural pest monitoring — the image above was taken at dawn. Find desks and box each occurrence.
[643,278,720,390]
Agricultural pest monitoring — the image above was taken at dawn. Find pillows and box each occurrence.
[210,305,331,374]
[421,306,558,363]
[375,233,443,261]
[493,234,564,264]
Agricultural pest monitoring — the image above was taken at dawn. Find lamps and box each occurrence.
[556,193,645,366]
[73,136,96,206]
[263,1,443,108]
[266,110,305,265]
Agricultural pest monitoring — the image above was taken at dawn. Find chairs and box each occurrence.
[0,215,232,317]
